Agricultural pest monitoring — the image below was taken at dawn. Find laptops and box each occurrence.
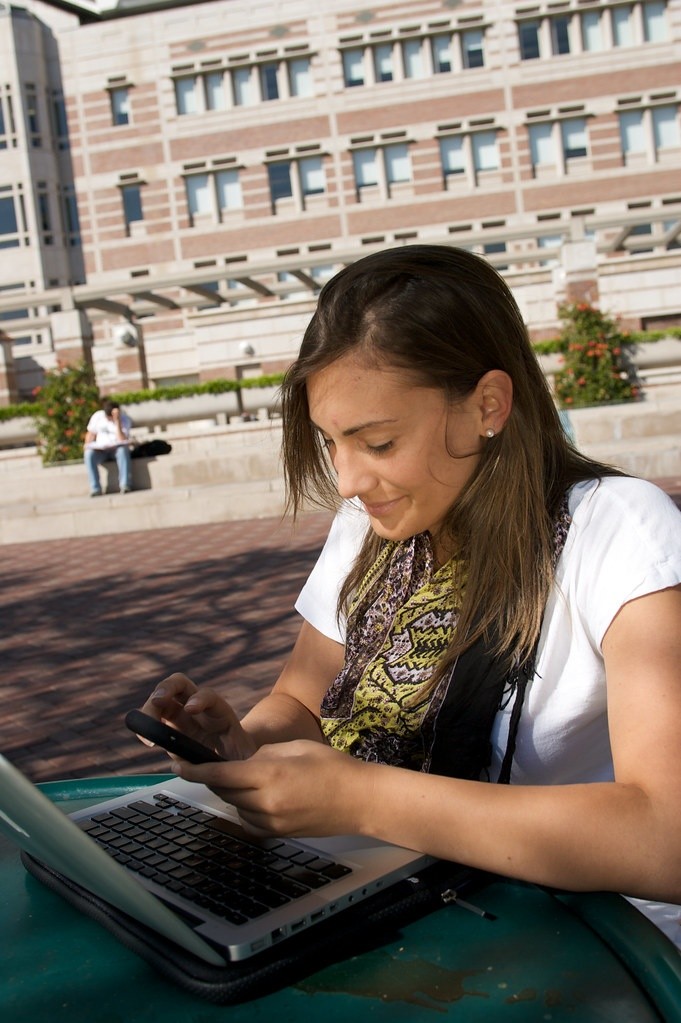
[0,754,440,964]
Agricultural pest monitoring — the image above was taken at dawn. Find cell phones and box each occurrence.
[124,709,227,766]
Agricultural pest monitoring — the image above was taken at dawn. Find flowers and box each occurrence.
[553,298,647,408]
[31,361,103,467]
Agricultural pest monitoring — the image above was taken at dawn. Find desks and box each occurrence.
[0,774,681,1023]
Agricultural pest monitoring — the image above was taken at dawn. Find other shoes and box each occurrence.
[88,489,101,496]
[119,488,129,494]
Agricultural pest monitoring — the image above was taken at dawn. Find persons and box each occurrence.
[82,398,135,495]
[124,245,681,952]
